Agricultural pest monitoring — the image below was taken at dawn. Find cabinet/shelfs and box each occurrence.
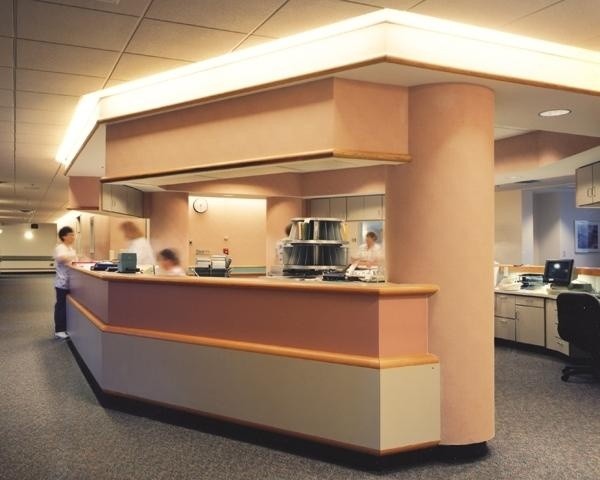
[280,215,349,279]
[308,195,387,222]
[574,162,600,208]
[66,176,144,218]
[494,291,600,359]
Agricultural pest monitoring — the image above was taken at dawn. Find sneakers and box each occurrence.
[55,331,70,340]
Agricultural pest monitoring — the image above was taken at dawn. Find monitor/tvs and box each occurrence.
[543,259,573,286]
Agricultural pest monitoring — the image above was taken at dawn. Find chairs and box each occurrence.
[555,291,600,379]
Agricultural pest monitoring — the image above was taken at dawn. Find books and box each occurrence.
[195,253,226,270]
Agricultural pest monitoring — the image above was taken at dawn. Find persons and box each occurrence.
[348,231,384,269]
[153,247,186,277]
[119,221,159,271]
[52,226,80,340]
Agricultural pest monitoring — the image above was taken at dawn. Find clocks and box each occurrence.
[192,198,207,211]
